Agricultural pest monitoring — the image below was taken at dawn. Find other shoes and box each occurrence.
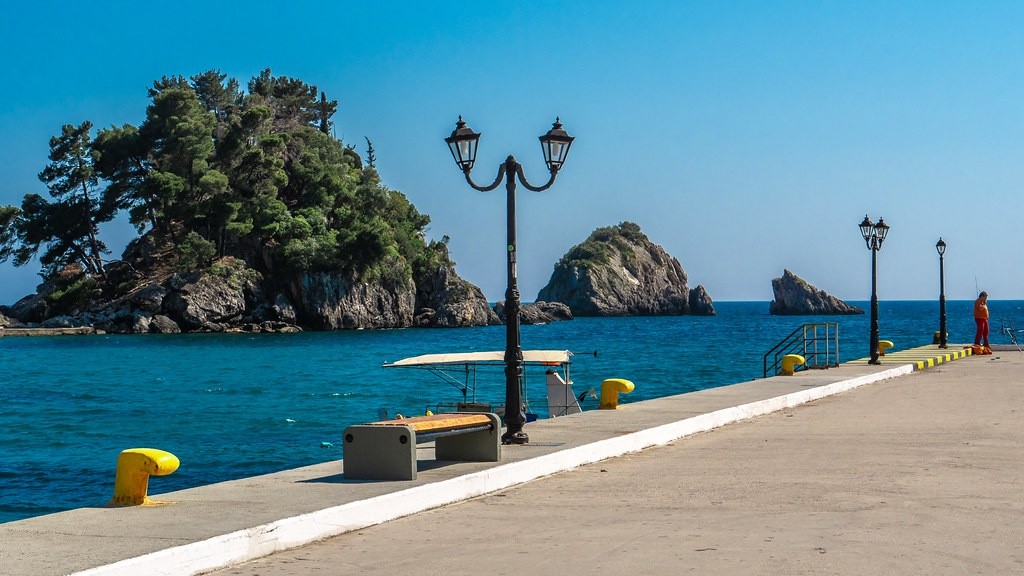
[983,345,992,349]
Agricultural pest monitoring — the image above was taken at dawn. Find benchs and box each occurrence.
[343,412,501,479]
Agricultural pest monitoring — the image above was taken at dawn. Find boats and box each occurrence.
[379,350,583,428]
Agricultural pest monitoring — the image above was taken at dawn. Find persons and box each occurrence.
[974,291,992,350]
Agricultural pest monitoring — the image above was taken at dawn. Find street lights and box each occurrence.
[936,236,950,349]
[443,113,575,442]
[858,213,890,364]
